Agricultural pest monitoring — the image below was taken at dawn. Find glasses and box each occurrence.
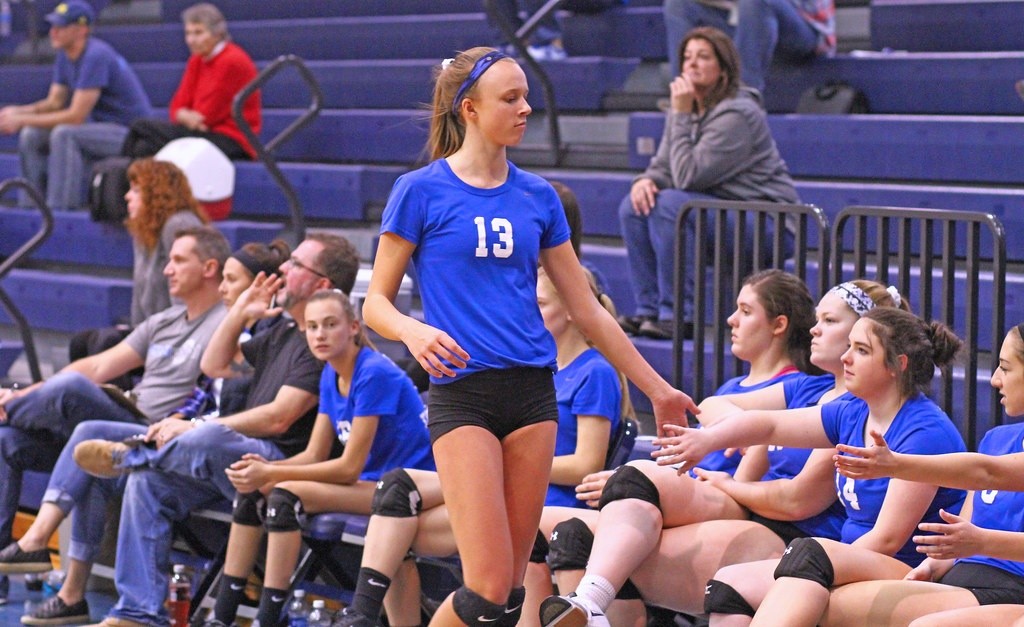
[290,258,335,285]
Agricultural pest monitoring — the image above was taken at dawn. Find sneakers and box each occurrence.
[82,617,147,627]
[74,439,131,479]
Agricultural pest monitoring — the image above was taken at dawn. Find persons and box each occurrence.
[0,0,154,210]
[615,26,801,339]
[656,1,838,114]
[0,159,1024,627]
[362,46,701,627]
[122,2,262,159]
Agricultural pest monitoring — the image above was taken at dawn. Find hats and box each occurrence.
[43,0,96,26]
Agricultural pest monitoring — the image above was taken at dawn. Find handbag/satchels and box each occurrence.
[797,78,872,115]
[89,154,133,223]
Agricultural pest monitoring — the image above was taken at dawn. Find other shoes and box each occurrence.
[617,312,657,336]
[332,607,379,627]
[538,591,611,627]
[21,595,90,627]
[639,320,694,339]
[0,541,54,573]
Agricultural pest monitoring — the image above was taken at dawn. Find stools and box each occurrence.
[183,497,367,627]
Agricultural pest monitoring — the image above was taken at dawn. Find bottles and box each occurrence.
[310,600,332,626]
[288,590,309,627]
[168,564,192,627]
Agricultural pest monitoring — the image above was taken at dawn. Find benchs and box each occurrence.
[0,0,1024,410]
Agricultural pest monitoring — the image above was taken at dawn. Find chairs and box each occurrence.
[411,418,638,627]
